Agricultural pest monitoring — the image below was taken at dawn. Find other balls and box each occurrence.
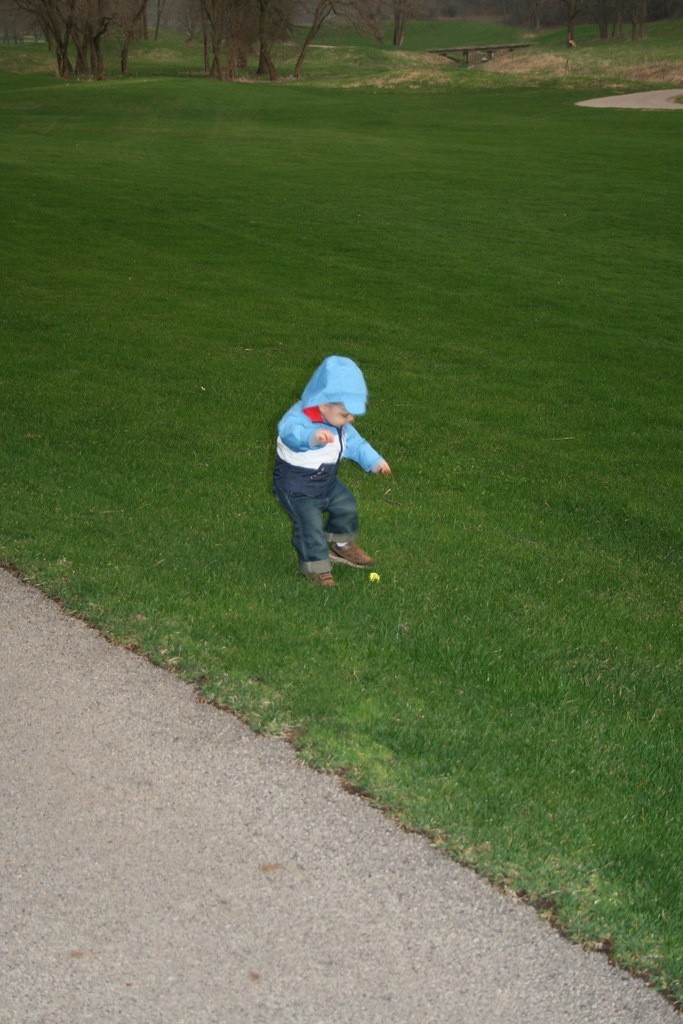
[369,571,382,583]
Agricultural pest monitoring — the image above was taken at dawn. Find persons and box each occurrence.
[273,355,390,586]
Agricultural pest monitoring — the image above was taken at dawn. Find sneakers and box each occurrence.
[308,572,335,587]
[327,541,374,569]
